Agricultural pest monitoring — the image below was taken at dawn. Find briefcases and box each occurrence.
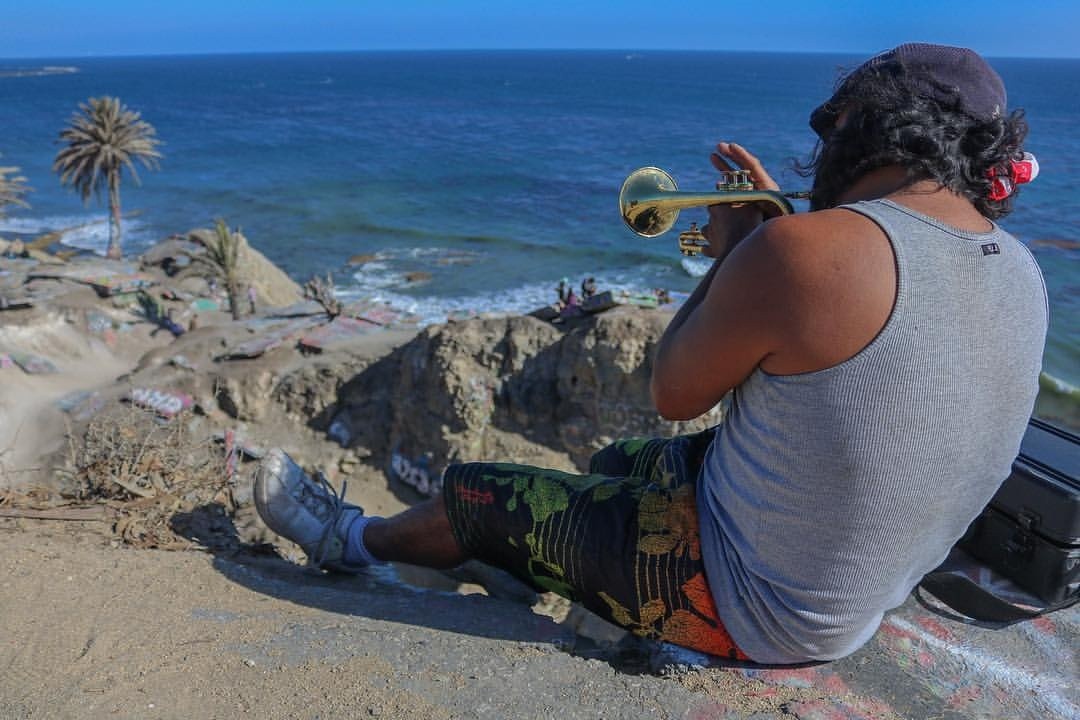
[920,418,1079,621]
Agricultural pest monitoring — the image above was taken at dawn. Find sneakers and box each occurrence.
[253,447,363,574]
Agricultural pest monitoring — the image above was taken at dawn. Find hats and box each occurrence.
[809,41,1008,141]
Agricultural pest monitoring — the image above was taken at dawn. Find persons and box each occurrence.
[559,279,568,308]
[253,43,1050,665]
[583,277,596,298]
[551,286,576,325]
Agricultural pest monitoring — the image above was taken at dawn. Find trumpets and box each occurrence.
[619,166,813,257]
